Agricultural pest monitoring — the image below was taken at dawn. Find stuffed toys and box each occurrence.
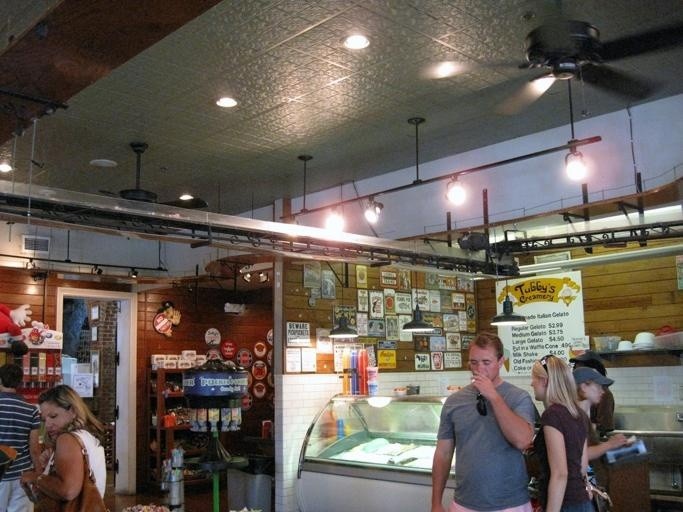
[0,303,33,356]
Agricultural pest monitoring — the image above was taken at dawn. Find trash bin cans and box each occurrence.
[227,460,275,512]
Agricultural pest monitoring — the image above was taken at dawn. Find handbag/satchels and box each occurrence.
[585,485,615,512]
[30,429,107,512]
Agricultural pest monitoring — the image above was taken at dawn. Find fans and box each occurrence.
[418,0,683,119]
[98,141,209,210]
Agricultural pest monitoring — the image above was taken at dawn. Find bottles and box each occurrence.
[342,346,376,395]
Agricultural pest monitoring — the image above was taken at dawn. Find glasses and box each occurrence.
[475,391,489,417]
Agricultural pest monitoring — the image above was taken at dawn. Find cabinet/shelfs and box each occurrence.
[0,328,63,404]
[147,368,228,496]
[297,394,457,512]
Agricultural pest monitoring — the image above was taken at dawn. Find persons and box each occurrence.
[0,362,42,512]
[573,366,628,461]
[530,353,596,512]
[568,350,615,430]
[19,384,108,512]
[430,330,536,512]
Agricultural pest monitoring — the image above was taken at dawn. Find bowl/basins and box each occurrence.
[394,390,406,396]
[591,336,622,353]
[445,389,459,396]
[615,332,657,352]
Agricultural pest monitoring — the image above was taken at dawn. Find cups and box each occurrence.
[365,367,379,395]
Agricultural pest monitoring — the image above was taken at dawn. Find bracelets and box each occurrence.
[36,474,45,486]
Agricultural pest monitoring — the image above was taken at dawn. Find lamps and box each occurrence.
[403,271,435,332]
[490,279,526,326]
[328,262,359,337]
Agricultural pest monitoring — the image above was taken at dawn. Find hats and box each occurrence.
[572,367,615,386]
[568,350,603,362]
